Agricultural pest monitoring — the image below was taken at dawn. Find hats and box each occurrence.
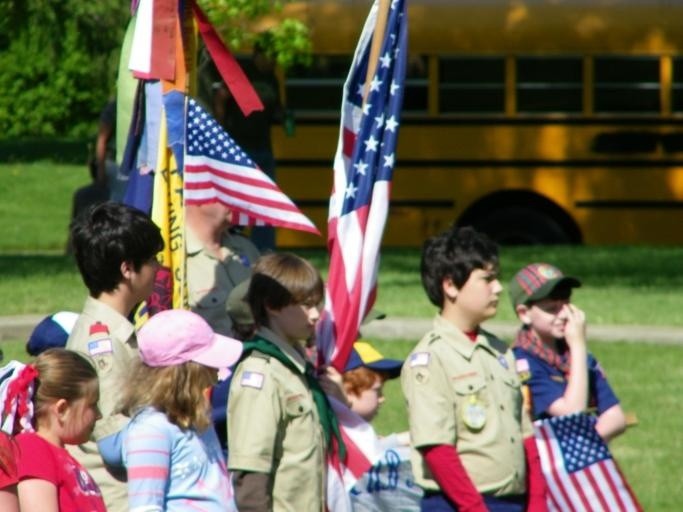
[509,262,583,314]
[137,310,243,369]
[344,342,403,380]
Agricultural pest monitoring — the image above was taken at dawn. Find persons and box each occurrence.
[509,263,646,512]
[228,254,332,512]
[185,201,252,342]
[63,203,165,512]
[12,349,108,512]
[123,308,246,512]
[324,342,421,511]
[216,57,278,235]
[0,429,21,512]
[93,97,131,203]
[26,312,83,357]
[399,226,548,512]
[70,159,111,255]
[210,279,261,458]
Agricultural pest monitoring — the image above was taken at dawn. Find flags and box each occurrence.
[319,0,408,369]
[164,93,323,249]
[532,414,641,512]
